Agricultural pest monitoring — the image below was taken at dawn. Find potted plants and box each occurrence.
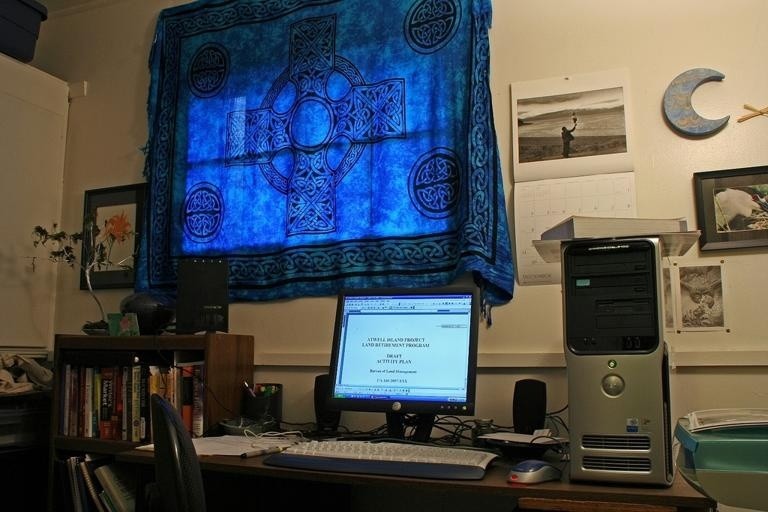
[20,223,138,334]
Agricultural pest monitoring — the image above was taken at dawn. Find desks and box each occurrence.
[121,436,716,512]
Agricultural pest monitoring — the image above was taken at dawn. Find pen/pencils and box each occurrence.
[242,381,257,400]
[240,447,282,459]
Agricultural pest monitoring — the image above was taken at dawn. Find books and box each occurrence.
[0,352,205,512]
[533,214,702,263]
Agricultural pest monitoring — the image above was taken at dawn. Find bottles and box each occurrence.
[470,417,496,458]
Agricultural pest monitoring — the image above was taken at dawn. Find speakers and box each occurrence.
[177,256,229,334]
[513,380,547,433]
[313,373,342,437]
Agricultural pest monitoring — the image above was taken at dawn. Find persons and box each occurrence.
[561,122,578,157]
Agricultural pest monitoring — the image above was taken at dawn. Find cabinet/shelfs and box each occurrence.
[46,336,254,512]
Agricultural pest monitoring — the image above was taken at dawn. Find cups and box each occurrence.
[246,382,282,430]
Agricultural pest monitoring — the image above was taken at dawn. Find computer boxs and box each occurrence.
[561,236,675,486]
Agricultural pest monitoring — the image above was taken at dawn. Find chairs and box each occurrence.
[153,393,207,512]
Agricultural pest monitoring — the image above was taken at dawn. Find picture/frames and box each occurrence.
[80,181,148,289]
[692,165,768,251]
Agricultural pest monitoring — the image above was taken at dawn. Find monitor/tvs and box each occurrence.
[325,286,481,437]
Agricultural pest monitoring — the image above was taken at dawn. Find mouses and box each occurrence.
[508,459,562,484]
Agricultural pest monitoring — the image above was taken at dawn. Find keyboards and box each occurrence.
[264,438,497,481]
